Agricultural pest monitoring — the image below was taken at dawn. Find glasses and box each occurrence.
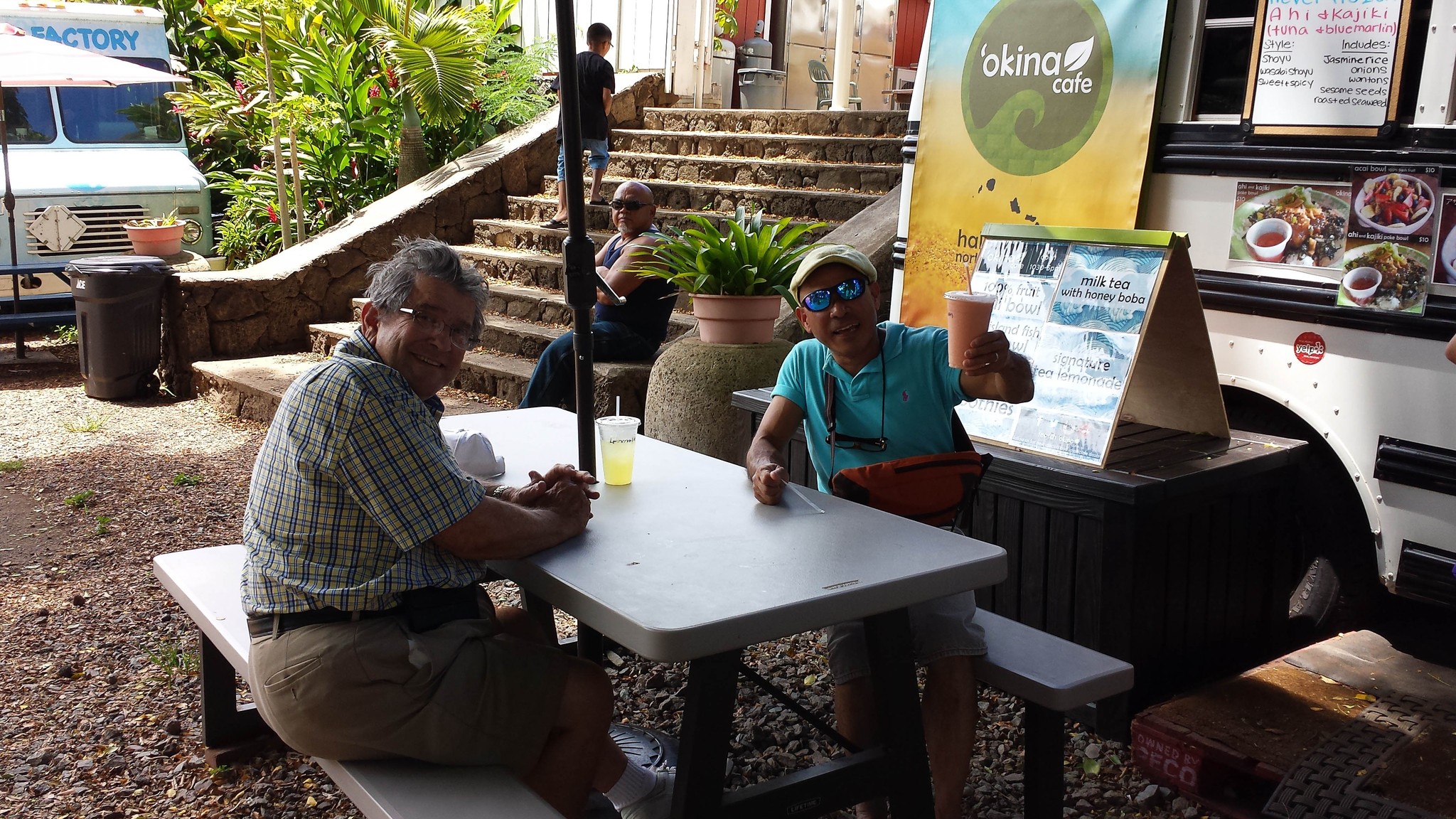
[612,200,651,211]
[825,432,889,452]
[801,279,869,312]
[396,308,481,351]
[603,41,613,49]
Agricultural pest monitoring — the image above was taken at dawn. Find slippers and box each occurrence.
[590,196,609,205]
[540,219,568,228]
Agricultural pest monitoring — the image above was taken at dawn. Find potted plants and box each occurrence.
[625,198,852,344]
[120,207,186,255]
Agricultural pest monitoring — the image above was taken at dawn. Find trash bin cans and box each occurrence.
[65,256,165,401]
[739,67,785,110]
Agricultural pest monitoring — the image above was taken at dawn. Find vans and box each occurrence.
[0,0,228,289]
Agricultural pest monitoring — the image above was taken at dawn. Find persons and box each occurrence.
[746,245,1035,819]
[518,181,680,414]
[539,23,615,229]
[239,232,743,819]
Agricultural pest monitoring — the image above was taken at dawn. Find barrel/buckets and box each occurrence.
[738,69,786,109]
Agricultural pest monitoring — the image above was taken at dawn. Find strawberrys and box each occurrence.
[1373,181,1430,224]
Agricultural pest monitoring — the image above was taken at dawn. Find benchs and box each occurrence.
[153,542,565,819]
[970,611,1135,819]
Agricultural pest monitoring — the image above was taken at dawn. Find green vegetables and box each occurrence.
[1258,186,1316,223]
[1353,241,1403,271]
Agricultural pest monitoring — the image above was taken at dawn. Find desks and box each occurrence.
[437,405,1007,819]
[1131,634,1456,819]
[882,89,913,111]
[725,385,1313,658]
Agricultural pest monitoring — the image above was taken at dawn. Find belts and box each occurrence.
[248,606,387,638]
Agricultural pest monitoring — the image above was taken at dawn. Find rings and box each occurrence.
[994,352,999,362]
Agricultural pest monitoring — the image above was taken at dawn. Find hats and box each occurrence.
[441,428,506,476]
[790,244,877,307]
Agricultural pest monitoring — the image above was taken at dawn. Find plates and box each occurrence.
[1231,188,1350,267]
[1354,174,1435,235]
[1337,243,1429,314]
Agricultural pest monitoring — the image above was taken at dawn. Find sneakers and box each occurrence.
[621,760,676,819]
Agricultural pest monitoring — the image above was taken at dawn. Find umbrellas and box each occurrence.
[0,21,192,359]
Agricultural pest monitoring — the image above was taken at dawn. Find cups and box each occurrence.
[16,128,27,141]
[943,290,997,369]
[596,416,641,485]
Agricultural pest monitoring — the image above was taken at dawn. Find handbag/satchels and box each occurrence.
[832,450,983,527]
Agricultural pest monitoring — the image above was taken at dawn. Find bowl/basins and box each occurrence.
[1441,225,1456,285]
[1343,267,1382,298]
[1246,218,1292,262]
[206,257,226,271]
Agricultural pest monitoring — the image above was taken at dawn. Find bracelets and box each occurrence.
[492,485,513,498]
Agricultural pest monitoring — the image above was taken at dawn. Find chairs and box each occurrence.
[808,60,862,110]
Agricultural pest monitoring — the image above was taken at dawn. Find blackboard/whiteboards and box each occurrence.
[1239,0,1410,138]
